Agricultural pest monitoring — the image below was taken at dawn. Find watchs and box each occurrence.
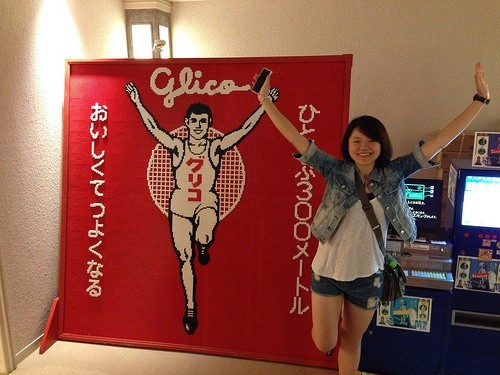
[470,92,493,107]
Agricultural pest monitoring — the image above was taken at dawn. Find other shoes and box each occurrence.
[324,349,334,356]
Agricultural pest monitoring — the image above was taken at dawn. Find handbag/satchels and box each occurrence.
[381,254,408,301]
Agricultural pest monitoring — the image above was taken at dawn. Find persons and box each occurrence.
[252,62,491,375]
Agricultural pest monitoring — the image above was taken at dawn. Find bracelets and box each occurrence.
[260,95,270,105]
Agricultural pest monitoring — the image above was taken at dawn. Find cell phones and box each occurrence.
[251,67,272,95]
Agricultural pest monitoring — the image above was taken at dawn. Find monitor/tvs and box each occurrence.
[453,168,500,250]
[387,178,444,237]
